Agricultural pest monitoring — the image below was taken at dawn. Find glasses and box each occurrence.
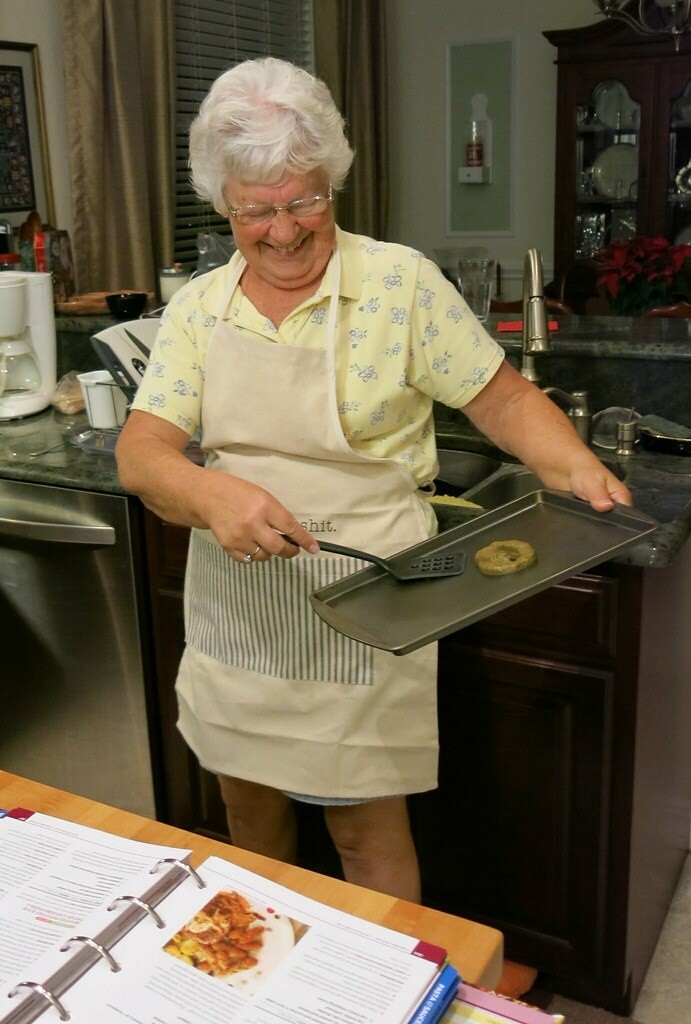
[218,182,338,222]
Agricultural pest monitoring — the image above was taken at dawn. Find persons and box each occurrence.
[116,55,632,913]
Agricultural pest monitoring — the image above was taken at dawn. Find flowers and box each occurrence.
[589,236,691,316]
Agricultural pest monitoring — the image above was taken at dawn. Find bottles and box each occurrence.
[1,219,15,254]
[0,254,22,271]
[465,119,486,166]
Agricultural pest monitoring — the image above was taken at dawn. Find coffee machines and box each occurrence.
[0,271,58,422]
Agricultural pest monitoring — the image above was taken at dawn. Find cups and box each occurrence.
[158,263,190,307]
[77,369,130,429]
[456,257,495,325]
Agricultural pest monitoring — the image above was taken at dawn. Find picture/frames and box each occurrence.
[446,35,522,241]
[0,39,58,233]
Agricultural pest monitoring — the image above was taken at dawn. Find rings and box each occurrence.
[242,545,260,563]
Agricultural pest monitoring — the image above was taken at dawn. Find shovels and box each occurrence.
[276,534,468,579]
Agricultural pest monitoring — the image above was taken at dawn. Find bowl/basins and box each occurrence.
[104,292,147,322]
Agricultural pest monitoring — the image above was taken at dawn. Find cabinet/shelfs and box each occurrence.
[541,0,690,315]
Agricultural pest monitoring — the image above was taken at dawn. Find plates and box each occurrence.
[572,74,691,253]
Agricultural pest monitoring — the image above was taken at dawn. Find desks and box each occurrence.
[1,774,503,1023]
[3,401,691,1014]
[468,312,691,425]
